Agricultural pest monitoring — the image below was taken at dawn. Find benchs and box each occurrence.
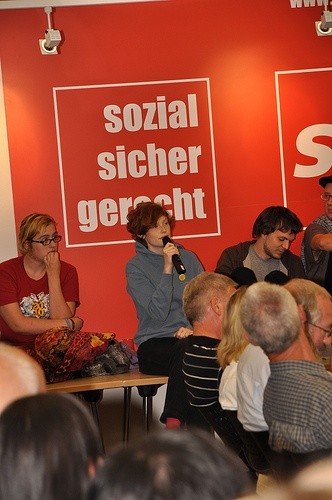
[46,369,168,458]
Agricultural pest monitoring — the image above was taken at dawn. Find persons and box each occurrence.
[126,202,205,431]
[0,213,84,346]
[0,175,332,500]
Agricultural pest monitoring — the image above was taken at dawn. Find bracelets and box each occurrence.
[65,318,75,331]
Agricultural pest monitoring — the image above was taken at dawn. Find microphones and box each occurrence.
[162,236,186,274]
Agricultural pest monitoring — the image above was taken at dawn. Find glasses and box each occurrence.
[320,194,332,201]
[305,321,332,337]
[30,236,62,246]
